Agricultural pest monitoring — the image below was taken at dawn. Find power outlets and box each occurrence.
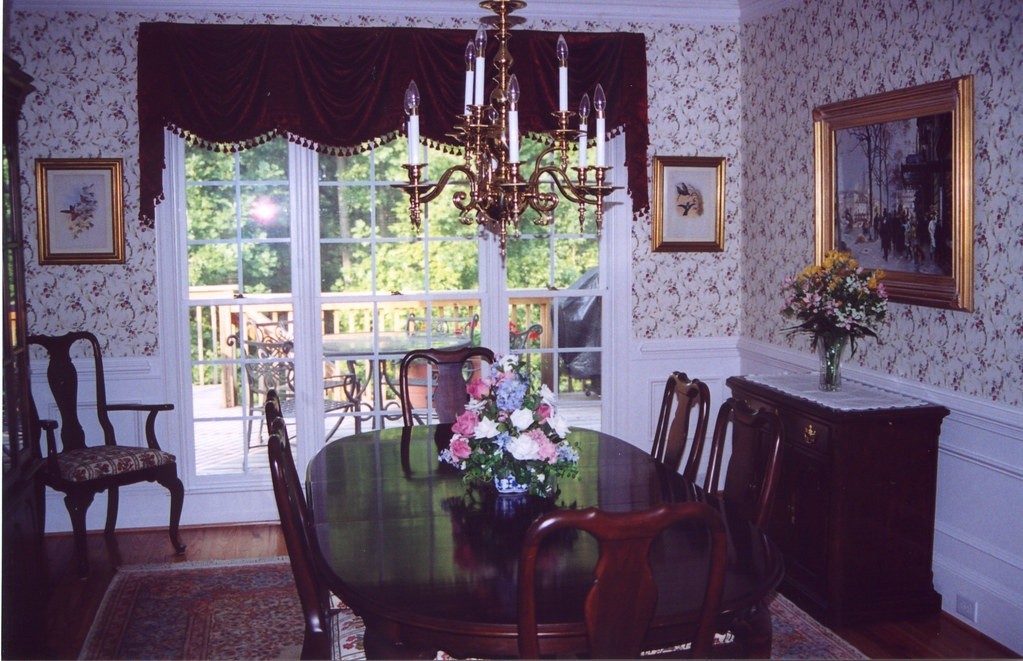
[956,593,979,624]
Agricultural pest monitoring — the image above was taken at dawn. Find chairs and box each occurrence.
[25,331,187,581]
[265,389,368,661]
[472,323,545,356]
[227,336,360,465]
[518,501,722,661]
[645,371,709,482]
[399,346,497,425]
[703,397,782,539]
[402,311,479,420]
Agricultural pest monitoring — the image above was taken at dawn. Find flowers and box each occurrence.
[778,251,889,358]
[436,354,587,499]
[402,309,544,355]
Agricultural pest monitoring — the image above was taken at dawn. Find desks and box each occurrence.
[305,332,473,442]
[308,425,791,661]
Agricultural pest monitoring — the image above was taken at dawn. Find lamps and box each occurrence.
[381,0,620,270]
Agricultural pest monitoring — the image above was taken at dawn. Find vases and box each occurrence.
[398,359,453,412]
[803,327,850,392]
[490,449,539,495]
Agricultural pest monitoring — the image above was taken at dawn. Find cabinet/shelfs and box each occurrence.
[2,55,44,661]
[725,371,944,621]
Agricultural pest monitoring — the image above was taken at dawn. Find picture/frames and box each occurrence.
[653,156,725,257]
[34,157,126,266]
[812,76,981,314]
